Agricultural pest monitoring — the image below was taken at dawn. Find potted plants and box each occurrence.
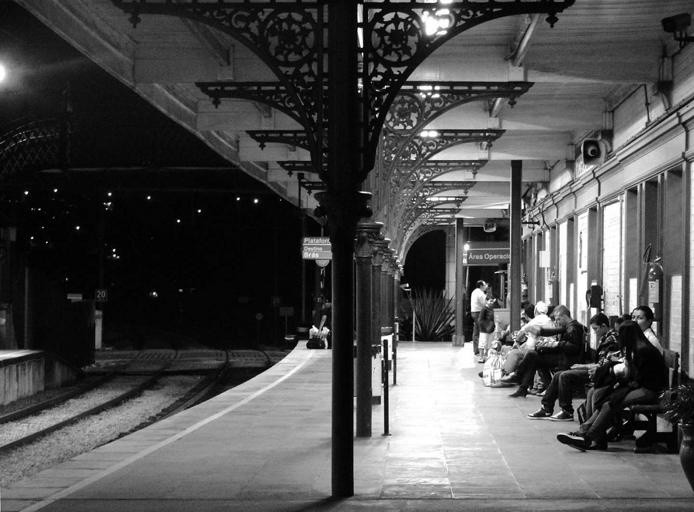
[658,384,694,491]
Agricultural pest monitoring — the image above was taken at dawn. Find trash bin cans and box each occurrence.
[352,344,382,405]
[381,327,393,370]
[394,316,399,342]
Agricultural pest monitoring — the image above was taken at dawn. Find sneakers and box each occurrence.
[527,405,574,421]
[499,372,547,398]
[557,429,624,451]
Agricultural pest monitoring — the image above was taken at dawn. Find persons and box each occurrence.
[317,302,332,350]
[470,279,497,355]
[476,287,668,452]
[307,307,322,341]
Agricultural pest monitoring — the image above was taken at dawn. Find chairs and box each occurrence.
[550,351,679,453]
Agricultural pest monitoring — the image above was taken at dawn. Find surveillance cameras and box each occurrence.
[660,13,692,33]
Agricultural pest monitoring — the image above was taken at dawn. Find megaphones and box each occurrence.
[483,222,497,233]
[580,137,606,166]
